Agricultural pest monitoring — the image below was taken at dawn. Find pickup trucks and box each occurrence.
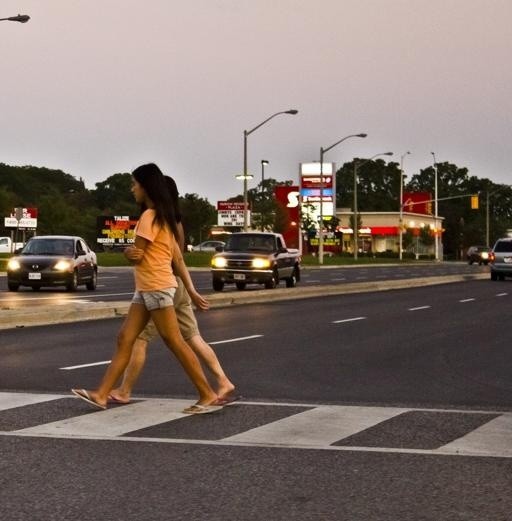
[211,232,301,291]
[1,237,26,255]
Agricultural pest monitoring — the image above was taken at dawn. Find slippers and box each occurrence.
[73,388,108,410]
[107,390,130,405]
[212,396,242,406]
[184,405,224,415]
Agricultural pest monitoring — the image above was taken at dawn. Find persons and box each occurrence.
[260,238,273,248]
[62,243,72,254]
[70,163,224,415]
[108,176,244,405]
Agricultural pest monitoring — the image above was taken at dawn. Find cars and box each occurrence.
[8,236,97,292]
[191,240,226,252]
[464,243,494,265]
[489,239,512,282]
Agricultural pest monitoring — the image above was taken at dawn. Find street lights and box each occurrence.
[233,109,298,232]
[319,133,367,265]
[352,151,510,263]
